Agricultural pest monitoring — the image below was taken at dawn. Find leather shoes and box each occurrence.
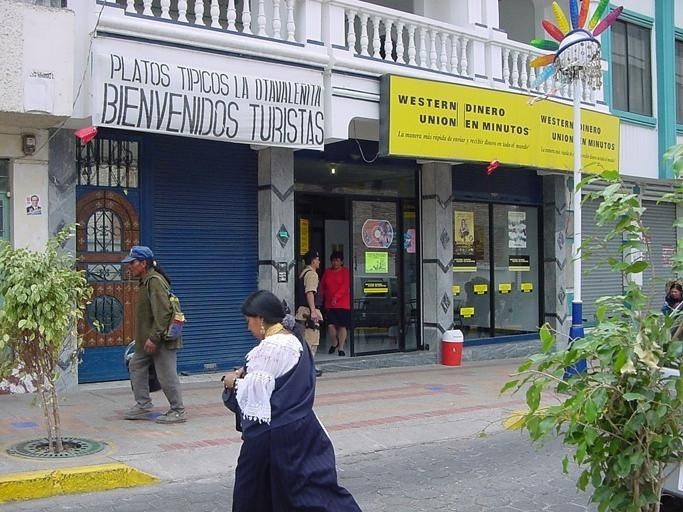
[328,339,339,354]
[338,349,345,357]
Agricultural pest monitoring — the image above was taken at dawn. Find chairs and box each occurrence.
[454,300,465,327]
[405,296,418,336]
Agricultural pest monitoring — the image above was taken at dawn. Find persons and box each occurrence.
[295,248,323,377]
[318,250,351,355]
[120,246,187,424]
[26,195,41,215]
[222,289,362,512]
[661,283,683,342]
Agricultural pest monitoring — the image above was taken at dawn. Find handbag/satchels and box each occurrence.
[161,292,187,342]
[124,339,161,393]
[222,366,245,440]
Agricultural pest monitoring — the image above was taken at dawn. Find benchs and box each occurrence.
[353,296,401,346]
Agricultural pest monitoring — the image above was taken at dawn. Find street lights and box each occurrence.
[557,30,600,394]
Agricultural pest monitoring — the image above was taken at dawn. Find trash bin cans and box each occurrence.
[441,329,464,366]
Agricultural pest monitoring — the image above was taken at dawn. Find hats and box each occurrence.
[120,245,154,264]
[303,247,320,260]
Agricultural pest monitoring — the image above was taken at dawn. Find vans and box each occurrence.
[352,273,399,309]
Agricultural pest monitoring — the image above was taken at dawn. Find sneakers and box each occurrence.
[124,400,155,418]
[154,407,188,424]
